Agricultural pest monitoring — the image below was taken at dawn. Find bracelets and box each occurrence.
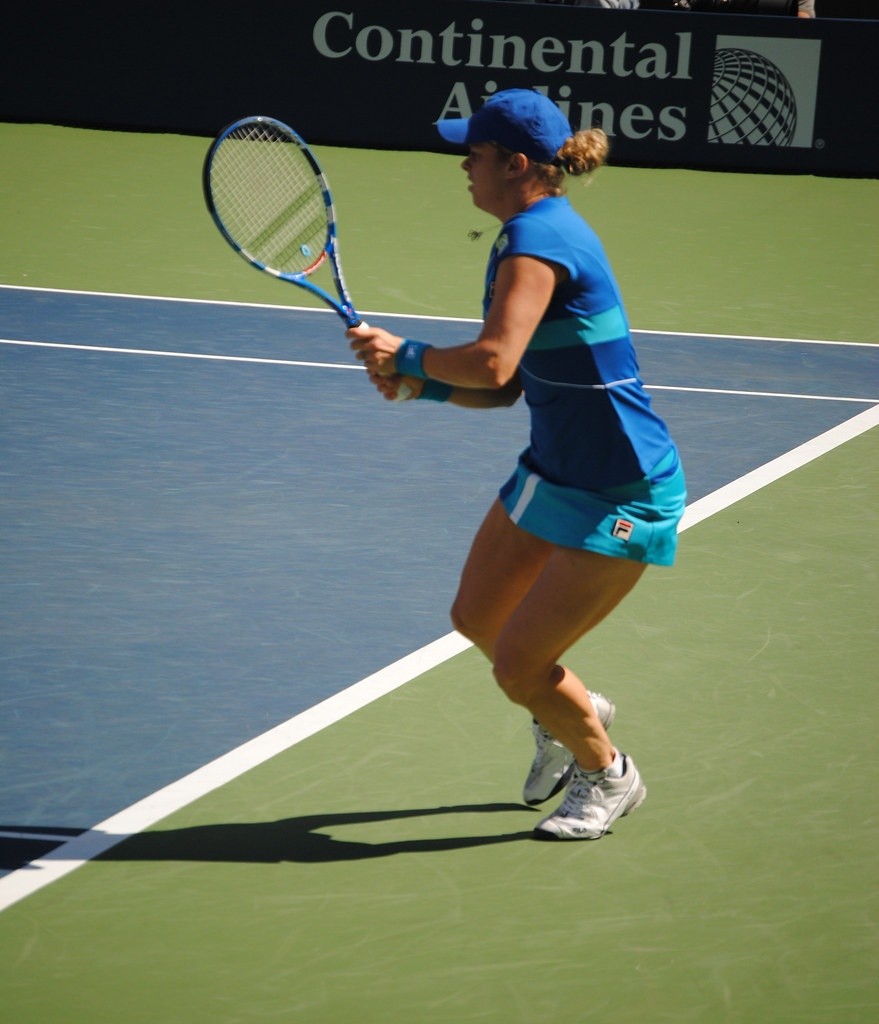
[395,338,432,382]
[416,377,452,403]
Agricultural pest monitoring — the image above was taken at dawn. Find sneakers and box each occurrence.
[533,755,647,841]
[523,692,615,806]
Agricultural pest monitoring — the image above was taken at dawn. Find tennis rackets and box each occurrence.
[203,116,412,402]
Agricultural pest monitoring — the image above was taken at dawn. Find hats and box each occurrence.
[437,88,573,168]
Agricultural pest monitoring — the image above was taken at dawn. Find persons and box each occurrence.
[575,0,817,19]
[346,89,687,840]
